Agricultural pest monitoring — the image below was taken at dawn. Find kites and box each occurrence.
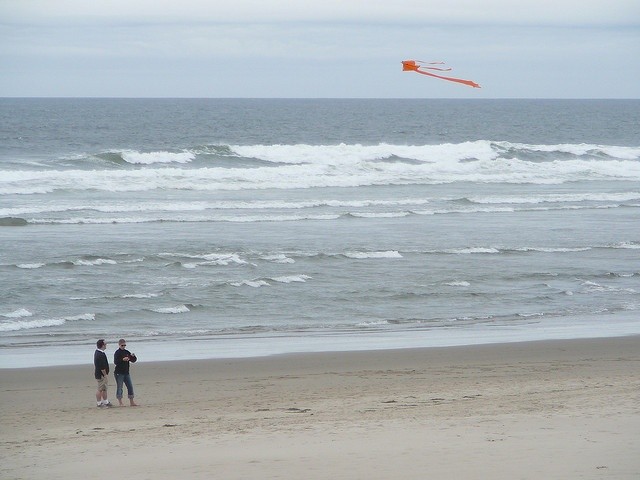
[401,60,482,89]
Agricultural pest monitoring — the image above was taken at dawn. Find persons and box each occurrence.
[94,339,115,408]
[114,339,139,406]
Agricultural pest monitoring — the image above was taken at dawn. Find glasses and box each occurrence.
[121,345,126,346]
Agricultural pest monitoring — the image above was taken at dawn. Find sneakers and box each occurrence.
[96,403,109,409]
[102,402,115,407]
